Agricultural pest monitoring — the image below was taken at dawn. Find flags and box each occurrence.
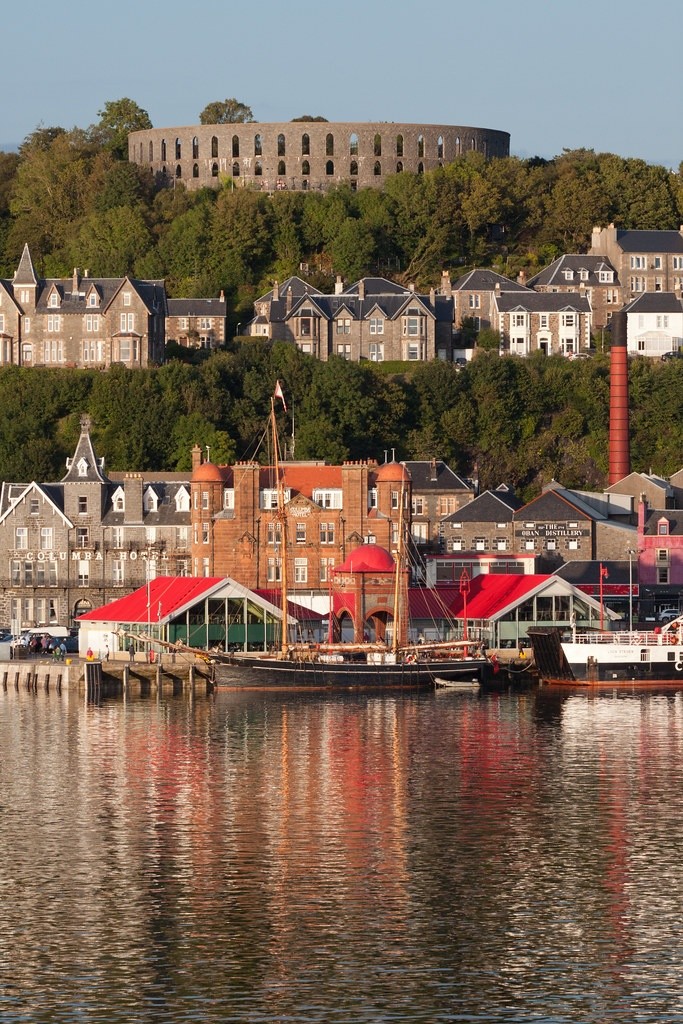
[276,381,287,412]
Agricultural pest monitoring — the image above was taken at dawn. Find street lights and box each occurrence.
[626,550,637,630]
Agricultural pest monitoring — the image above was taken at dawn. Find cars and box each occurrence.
[4,633,30,659]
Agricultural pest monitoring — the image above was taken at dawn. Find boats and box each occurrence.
[525,550,683,686]
[433,677,481,687]
[109,396,488,691]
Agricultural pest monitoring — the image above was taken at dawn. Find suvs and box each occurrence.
[658,608,682,624]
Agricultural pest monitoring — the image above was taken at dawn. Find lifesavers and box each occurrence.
[675,661,683,671]
[407,654,416,663]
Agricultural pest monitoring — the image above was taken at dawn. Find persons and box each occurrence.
[129,644,135,662]
[87,647,93,657]
[105,645,110,662]
[30,634,67,663]
